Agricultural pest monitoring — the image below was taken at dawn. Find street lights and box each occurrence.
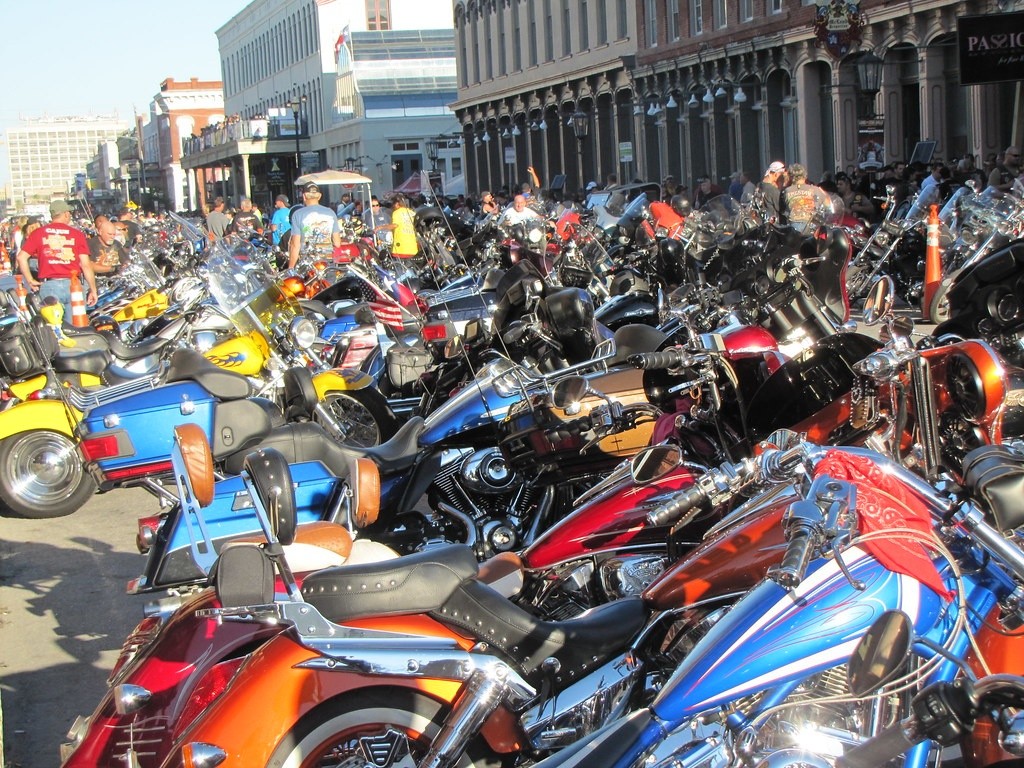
[291,97,302,177]
[135,161,142,205]
[425,141,439,171]
[571,111,588,189]
[854,51,884,201]
[345,156,356,202]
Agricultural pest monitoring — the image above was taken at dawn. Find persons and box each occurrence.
[0,147,1024,332]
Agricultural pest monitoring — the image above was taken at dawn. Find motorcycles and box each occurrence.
[0,179,1023,767]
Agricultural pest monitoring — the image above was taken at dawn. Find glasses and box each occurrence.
[1008,153,1020,158]
[372,203,379,207]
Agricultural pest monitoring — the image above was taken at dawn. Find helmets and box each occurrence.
[670,195,691,216]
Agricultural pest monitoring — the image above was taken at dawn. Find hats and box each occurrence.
[49,200,74,217]
[276,194,291,207]
[764,161,785,177]
[303,181,320,192]
[117,206,130,215]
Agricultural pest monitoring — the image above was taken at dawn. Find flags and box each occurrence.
[352,271,403,330]
[374,265,418,306]
